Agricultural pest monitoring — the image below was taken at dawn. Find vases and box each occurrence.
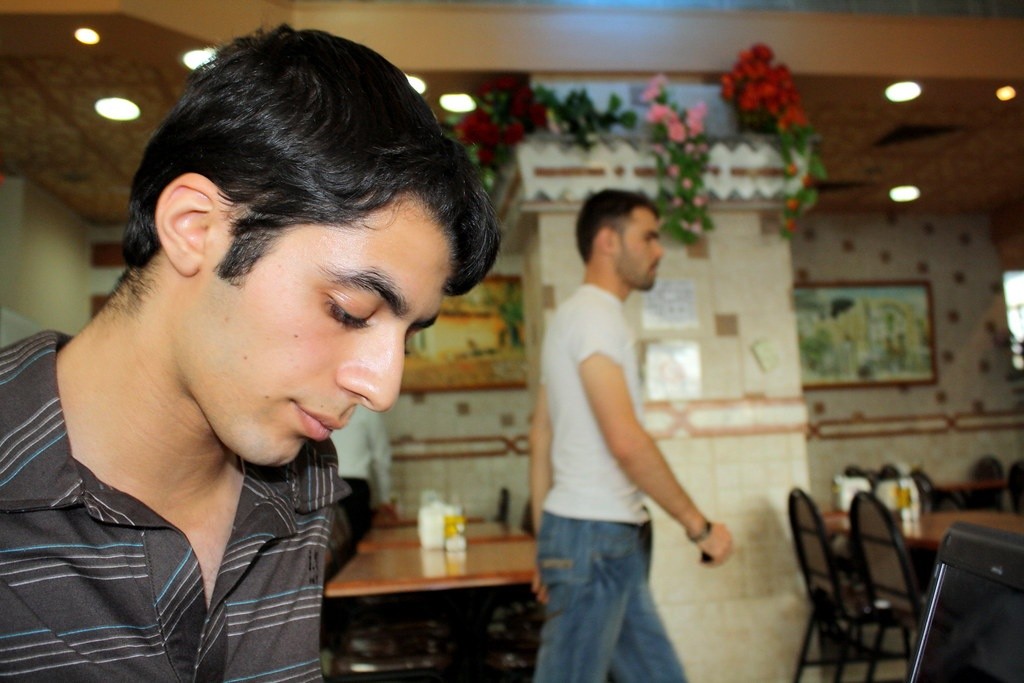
[737,87,777,132]
[517,133,809,202]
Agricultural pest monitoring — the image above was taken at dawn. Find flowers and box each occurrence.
[447,73,637,192]
[643,75,715,244]
[722,43,823,237]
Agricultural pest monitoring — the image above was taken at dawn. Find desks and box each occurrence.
[324,513,537,683]
[928,479,1009,493]
[804,510,1024,683]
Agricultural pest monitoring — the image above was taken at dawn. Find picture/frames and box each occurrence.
[400,274,526,392]
[795,281,939,390]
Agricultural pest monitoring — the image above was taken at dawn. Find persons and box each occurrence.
[0,24,502,683]
[529,188,735,683]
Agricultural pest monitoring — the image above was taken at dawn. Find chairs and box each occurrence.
[522,502,533,538]
[788,456,1023,683]
[496,488,508,521]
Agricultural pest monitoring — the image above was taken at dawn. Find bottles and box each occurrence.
[444,497,468,552]
[897,476,921,520]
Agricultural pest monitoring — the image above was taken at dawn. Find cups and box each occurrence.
[832,474,901,513]
[418,500,464,549]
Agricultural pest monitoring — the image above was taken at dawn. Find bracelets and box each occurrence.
[688,522,711,543]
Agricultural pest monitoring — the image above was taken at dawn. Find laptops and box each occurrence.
[907,520,1024,683]
